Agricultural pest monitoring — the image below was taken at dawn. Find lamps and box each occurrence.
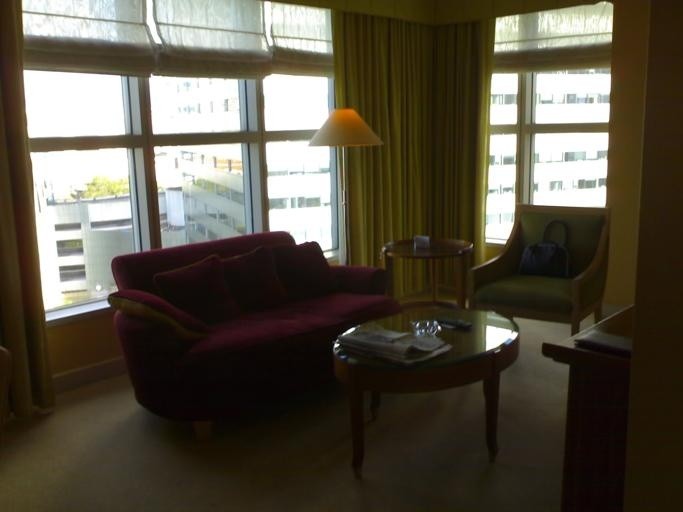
[311,110,384,261]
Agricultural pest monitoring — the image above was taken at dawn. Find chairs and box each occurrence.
[468,203,611,339]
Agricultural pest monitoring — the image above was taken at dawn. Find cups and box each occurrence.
[416,319,438,337]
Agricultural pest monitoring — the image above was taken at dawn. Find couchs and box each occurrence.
[109,232,404,423]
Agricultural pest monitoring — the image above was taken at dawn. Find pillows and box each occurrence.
[154,242,336,323]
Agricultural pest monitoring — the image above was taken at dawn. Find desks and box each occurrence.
[378,237,473,311]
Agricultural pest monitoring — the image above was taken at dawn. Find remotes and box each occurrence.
[438,318,472,329]
